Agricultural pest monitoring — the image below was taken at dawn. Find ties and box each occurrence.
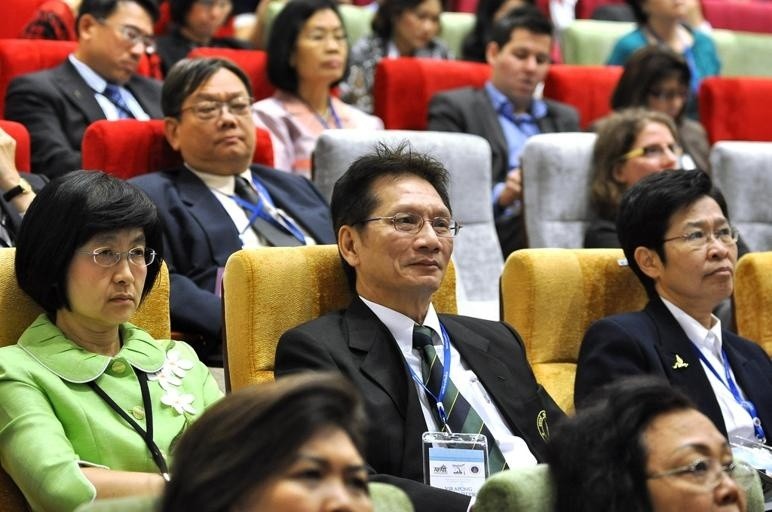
[408,322,516,480]
[229,176,308,249]
[104,84,141,122]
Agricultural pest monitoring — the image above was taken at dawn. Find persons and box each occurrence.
[249,0,386,181]
[426,7,581,263]
[155,0,256,80]
[590,0,713,29]
[461,0,564,64]
[0,128,52,248]
[546,375,748,512]
[154,369,374,512]
[124,56,337,367]
[338,0,456,114]
[5,0,164,178]
[606,0,721,89]
[0,169,227,512]
[274,139,571,512]
[573,167,772,512]
[582,107,753,332]
[588,44,711,175]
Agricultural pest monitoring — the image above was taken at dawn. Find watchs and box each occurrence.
[3,179,32,202]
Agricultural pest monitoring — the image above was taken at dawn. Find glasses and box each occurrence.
[646,87,689,102]
[659,225,742,251]
[611,139,687,162]
[349,210,462,238]
[72,241,159,269]
[95,15,160,52]
[171,96,259,121]
[643,455,758,492]
